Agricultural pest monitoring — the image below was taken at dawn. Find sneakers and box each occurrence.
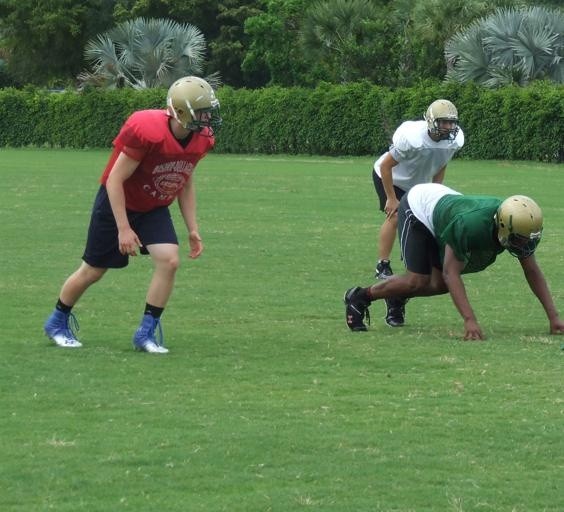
[344,287,371,333]
[385,295,411,328]
[375,260,397,280]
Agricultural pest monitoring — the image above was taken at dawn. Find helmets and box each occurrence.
[493,197,544,257]
[424,99,461,142]
[167,76,224,136]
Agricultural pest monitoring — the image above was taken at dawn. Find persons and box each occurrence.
[370,99,463,280]
[341,180,564,345]
[43,76,223,353]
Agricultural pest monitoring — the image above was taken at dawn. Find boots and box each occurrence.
[44,308,85,349]
[134,314,175,355]
